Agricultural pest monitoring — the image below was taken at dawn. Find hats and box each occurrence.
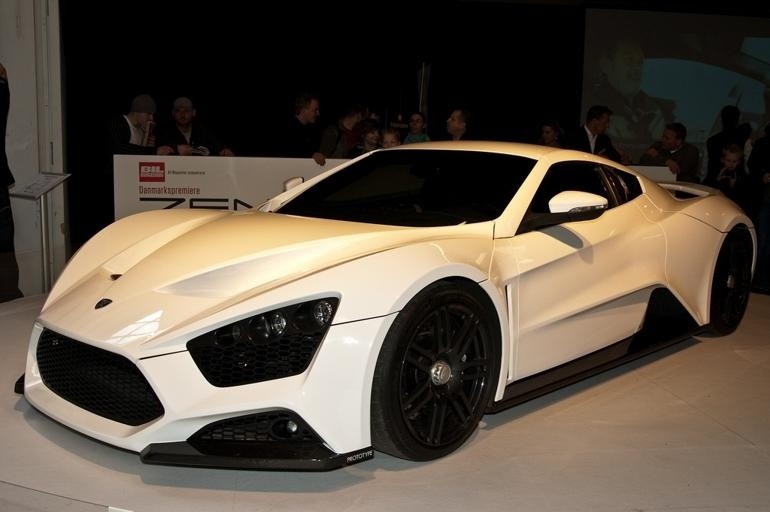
[173,97,193,108]
[130,94,156,114]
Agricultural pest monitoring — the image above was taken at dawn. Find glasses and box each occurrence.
[408,119,422,123]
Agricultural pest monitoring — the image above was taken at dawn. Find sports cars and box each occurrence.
[13,138,759,472]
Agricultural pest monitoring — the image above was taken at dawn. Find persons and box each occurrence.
[262,92,493,165]
[639,122,699,184]
[162,97,234,156]
[539,106,622,162]
[706,106,768,293]
[0,65,16,255]
[94,94,193,229]
